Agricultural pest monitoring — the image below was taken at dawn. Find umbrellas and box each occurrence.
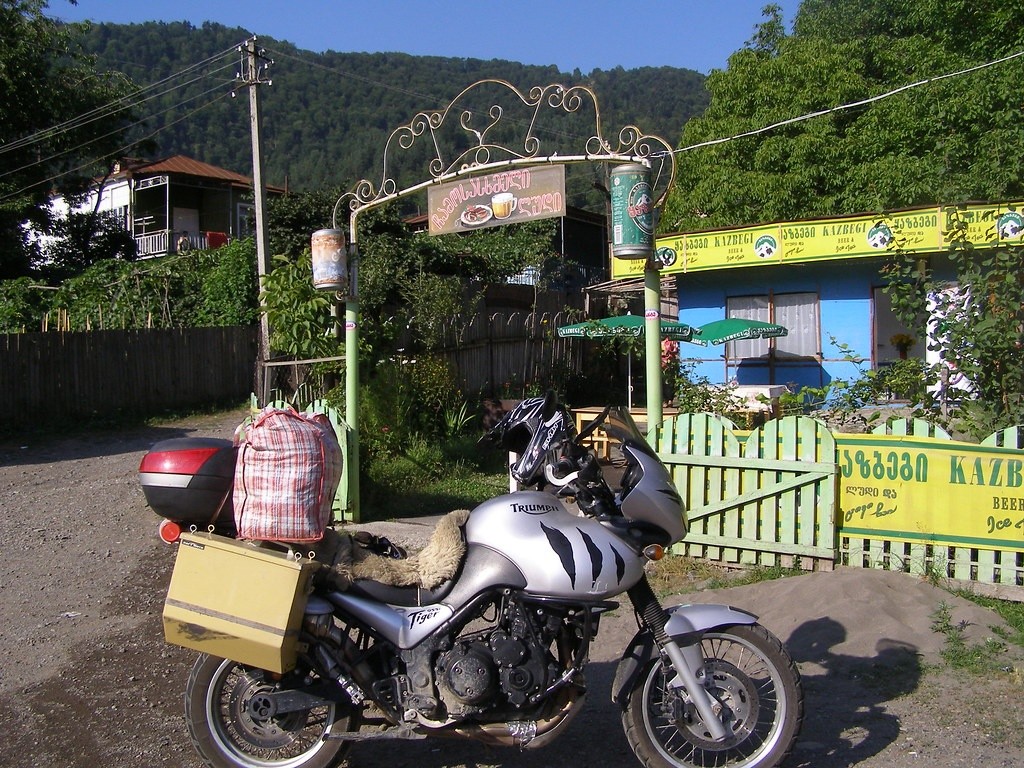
[688,315,788,381]
[557,311,702,412]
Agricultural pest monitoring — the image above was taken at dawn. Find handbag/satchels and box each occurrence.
[233,405,343,545]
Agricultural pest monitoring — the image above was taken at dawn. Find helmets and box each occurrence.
[498,397,578,487]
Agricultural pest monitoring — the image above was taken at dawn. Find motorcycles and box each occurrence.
[139,385,807,768]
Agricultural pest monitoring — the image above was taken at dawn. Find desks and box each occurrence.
[682,384,791,424]
[571,406,678,461]
